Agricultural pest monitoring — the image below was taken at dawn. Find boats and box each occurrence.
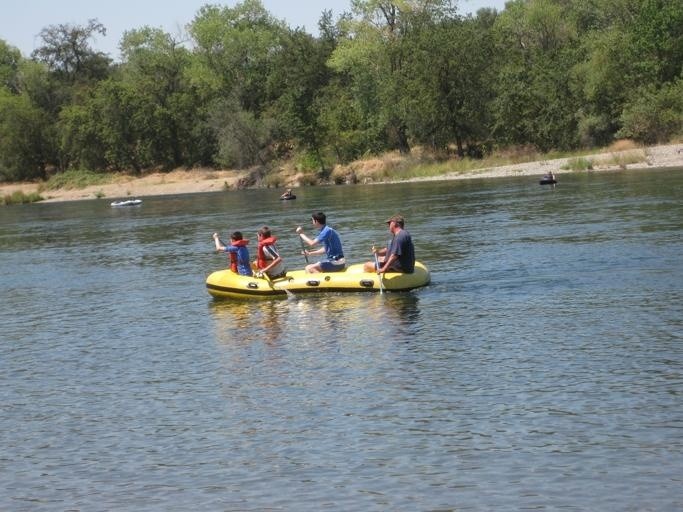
[283,195,296,200]
[111,200,142,206]
[205,261,430,299]
[540,180,557,185]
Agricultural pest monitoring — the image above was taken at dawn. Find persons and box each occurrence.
[295,212,346,273]
[212,231,253,277]
[254,224,287,278]
[363,213,416,273]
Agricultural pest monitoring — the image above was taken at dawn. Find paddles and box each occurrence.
[218,238,257,272]
[254,262,274,285]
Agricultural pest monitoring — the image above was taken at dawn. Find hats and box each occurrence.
[386,214,404,224]
